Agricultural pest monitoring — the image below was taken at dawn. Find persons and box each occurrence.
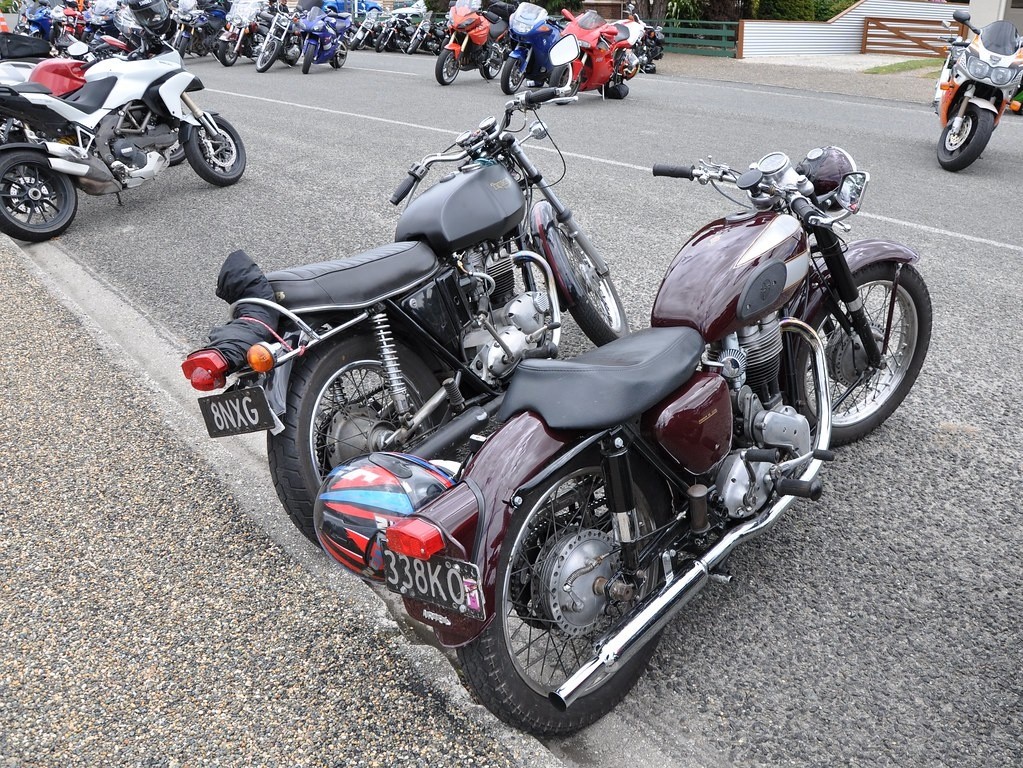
[269,0,289,12]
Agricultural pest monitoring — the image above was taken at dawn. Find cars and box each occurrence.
[391,0,427,17]
[320,0,382,14]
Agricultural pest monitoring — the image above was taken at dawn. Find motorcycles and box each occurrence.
[434,0,519,85]
[179,84,631,550]
[27,34,135,96]
[364,145,933,737]
[0,2,247,242]
[549,9,641,105]
[256,0,302,73]
[171,1,232,62]
[375,6,418,53]
[931,9,1023,172]
[622,4,665,74]
[10,0,172,42]
[500,1,565,94]
[406,10,449,54]
[291,1,352,74]
[349,3,384,51]
[219,0,270,67]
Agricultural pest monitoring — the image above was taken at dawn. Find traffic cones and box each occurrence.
[0,10,9,32]
